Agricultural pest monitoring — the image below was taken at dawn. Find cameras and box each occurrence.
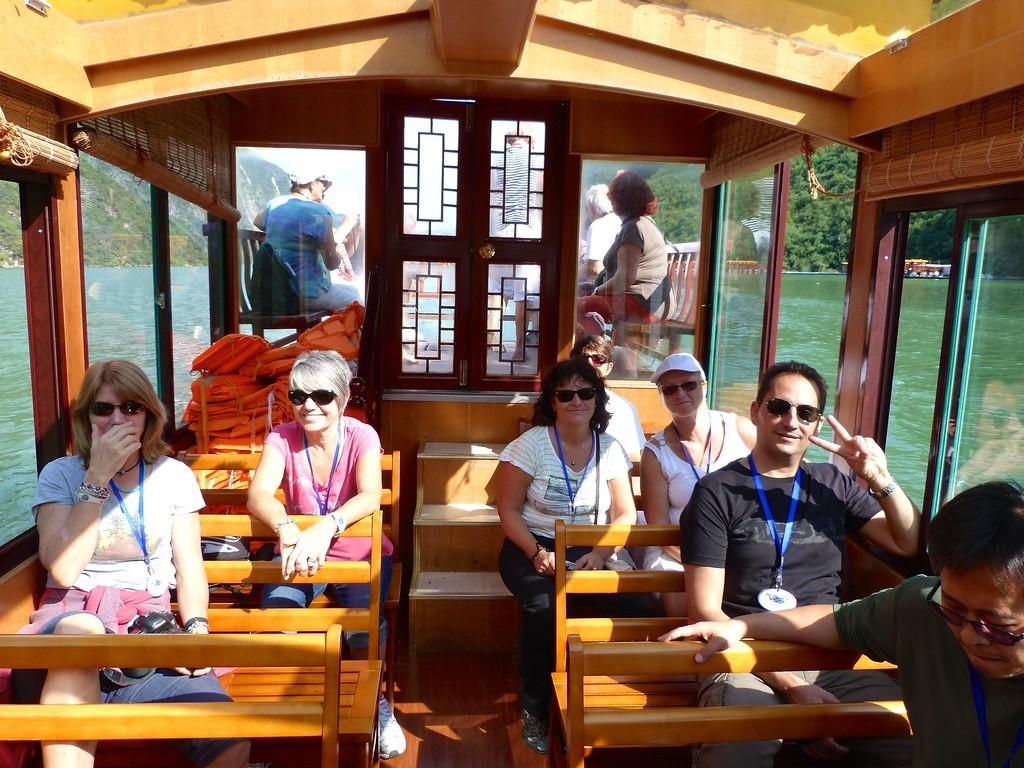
[118,609,194,678]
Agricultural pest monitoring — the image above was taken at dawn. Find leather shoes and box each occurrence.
[520,708,548,754]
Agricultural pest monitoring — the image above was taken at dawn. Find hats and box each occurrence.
[649,353,704,385]
[288,164,333,192]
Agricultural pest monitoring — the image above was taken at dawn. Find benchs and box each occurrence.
[203,224,332,349]
[551,518,912,768]
[0,450,403,768]
[611,240,702,361]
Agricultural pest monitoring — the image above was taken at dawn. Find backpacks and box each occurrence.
[249,241,304,315]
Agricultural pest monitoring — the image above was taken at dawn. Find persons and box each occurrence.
[569,334,649,571]
[939,368,1024,504]
[251,126,678,379]
[244,350,407,759]
[497,358,661,754]
[8,358,253,768]
[678,359,922,768]
[640,353,757,640]
[655,479,1024,768]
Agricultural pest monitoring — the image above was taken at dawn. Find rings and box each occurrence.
[593,567,597,570]
[310,557,317,564]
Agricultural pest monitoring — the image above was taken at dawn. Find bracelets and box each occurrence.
[954,476,973,491]
[184,617,210,633]
[530,543,545,561]
[274,518,296,533]
[869,479,899,499]
[75,481,109,505]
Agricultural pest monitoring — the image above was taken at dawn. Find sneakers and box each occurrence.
[376,692,407,760]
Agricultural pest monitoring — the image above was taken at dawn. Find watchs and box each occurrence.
[331,512,346,538]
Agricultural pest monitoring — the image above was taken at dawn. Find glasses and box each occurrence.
[348,377,367,409]
[661,379,703,395]
[87,399,143,416]
[288,388,337,405]
[757,398,822,424]
[926,578,1024,647]
[577,351,609,364]
[552,387,598,403]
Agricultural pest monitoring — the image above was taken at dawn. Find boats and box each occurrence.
[842,257,949,279]
[669,259,765,274]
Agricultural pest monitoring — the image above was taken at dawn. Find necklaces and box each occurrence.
[114,455,146,493]
[560,431,587,466]
